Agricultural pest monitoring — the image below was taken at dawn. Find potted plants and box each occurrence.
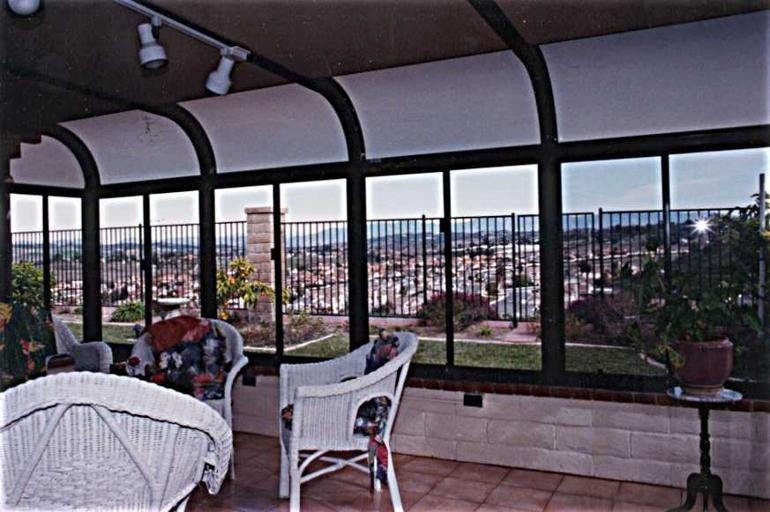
[615,240,764,398]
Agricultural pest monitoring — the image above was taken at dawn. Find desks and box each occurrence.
[666,384,745,511]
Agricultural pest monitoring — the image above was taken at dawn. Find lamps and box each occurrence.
[135,14,248,97]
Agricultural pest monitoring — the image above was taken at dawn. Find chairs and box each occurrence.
[121,318,249,483]
[53,317,115,374]
[1,368,237,510]
[278,329,423,512]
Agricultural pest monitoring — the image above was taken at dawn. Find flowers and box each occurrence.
[0,264,58,378]
